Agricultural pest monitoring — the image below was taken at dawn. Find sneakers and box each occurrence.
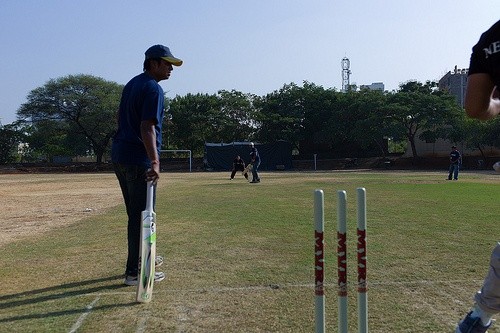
[455,309,493,333]
[124,271,165,286]
[155,256,163,267]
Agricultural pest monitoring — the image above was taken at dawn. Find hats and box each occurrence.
[145,44,183,66]
[249,142,254,145]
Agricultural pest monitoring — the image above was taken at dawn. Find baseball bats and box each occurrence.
[240,163,252,176]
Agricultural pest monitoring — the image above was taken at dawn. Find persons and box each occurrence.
[454,19,499,333]
[229,155,250,181]
[112,44,183,285]
[248,142,261,183]
[446,146,460,180]
